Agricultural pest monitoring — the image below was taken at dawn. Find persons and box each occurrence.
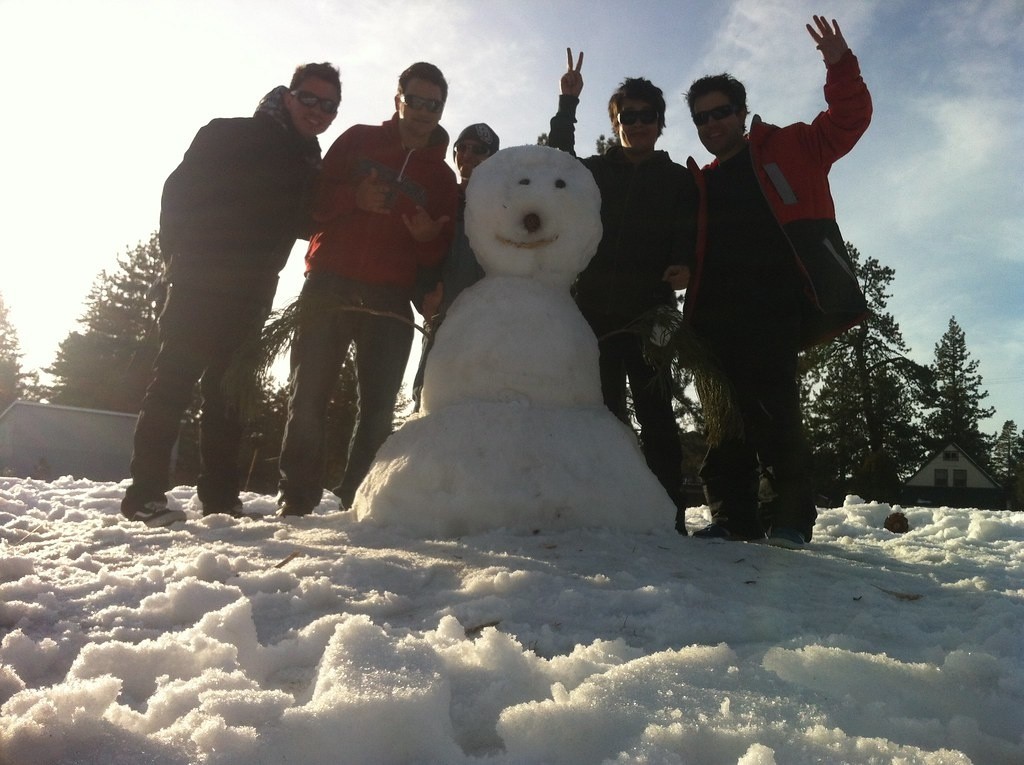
[121,61,340,525]
[275,61,458,517]
[546,46,698,538]
[412,123,500,415]
[686,15,874,546]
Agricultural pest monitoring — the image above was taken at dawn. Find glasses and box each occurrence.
[693,104,740,126]
[454,143,490,156]
[290,90,337,114]
[399,94,444,113]
[617,110,660,125]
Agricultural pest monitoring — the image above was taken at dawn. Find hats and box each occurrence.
[453,123,499,163]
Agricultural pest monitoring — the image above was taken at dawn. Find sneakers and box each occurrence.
[694,523,763,544]
[767,525,805,550]
[205,506,263,523]
[131,501,187,528]
